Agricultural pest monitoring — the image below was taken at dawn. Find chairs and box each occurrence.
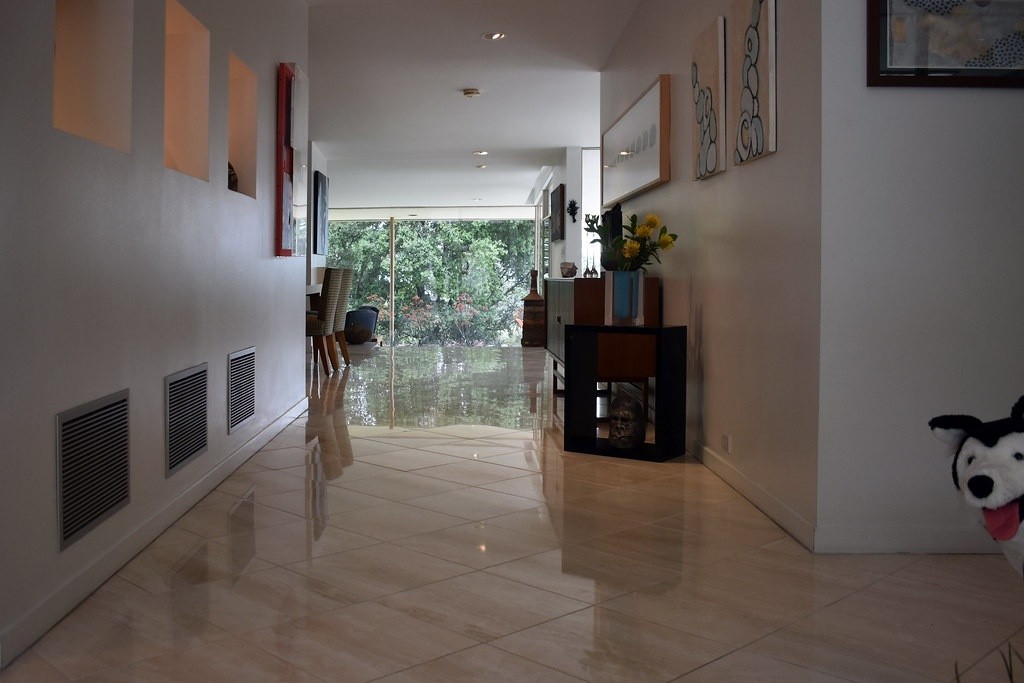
[306,266,343,378]
[313,268,354,370]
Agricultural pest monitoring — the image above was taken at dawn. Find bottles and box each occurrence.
[591,257,598,278]
[584,257,591,278]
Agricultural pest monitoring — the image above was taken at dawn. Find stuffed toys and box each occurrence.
[929,395,1024,579]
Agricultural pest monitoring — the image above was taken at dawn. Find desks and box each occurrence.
[306,283,323,296]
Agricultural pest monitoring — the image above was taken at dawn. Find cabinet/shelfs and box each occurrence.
[543,275,661,425]
[564,324,687,463]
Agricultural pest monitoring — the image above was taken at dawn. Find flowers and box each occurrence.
[583,204,678,273]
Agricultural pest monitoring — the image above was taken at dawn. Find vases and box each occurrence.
[613,271,639,326]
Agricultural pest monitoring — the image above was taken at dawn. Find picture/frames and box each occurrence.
[867,0,1024,89]
[600,73,670,210]
[315,170,329,255]
[550,183,565,242]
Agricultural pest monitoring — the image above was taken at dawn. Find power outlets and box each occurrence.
[721,433,731,455]
